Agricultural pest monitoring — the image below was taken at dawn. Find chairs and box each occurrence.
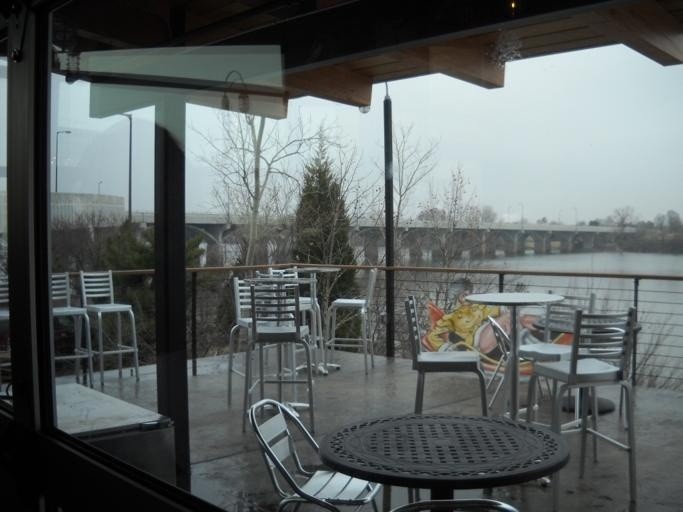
[0,272,140,393]
